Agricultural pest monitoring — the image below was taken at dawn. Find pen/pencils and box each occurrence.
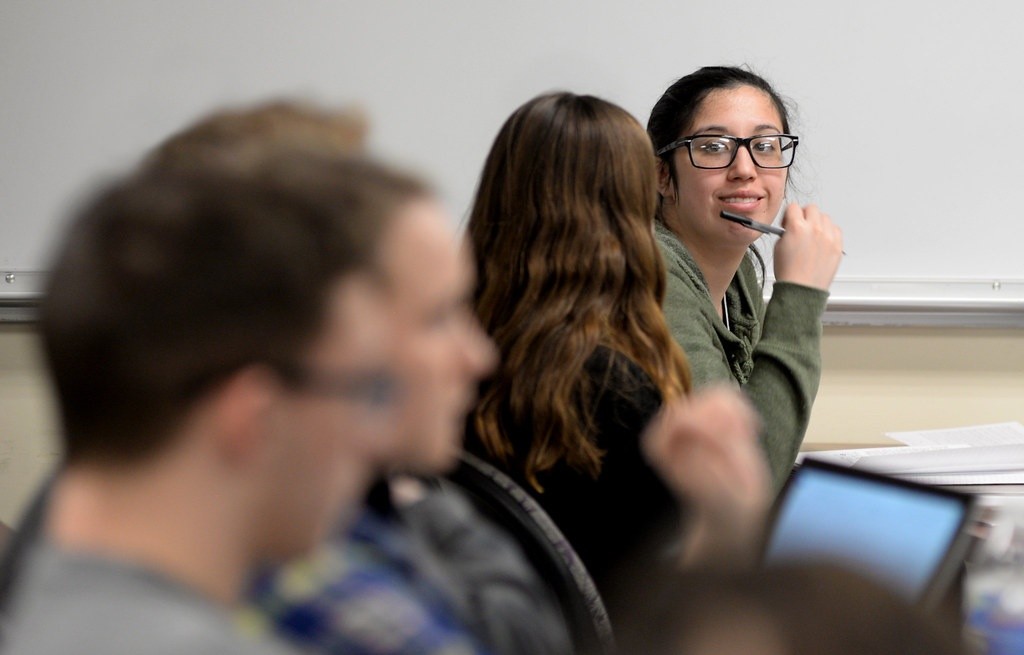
[720,209,784,237]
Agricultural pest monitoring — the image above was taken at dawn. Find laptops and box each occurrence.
[758,457,977,607]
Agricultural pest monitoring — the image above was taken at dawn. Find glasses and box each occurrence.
[655,134,799,169]
[264,357,401,420]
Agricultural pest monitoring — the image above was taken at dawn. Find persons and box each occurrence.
[1,100,499,655]
[644,66,844,525]
[454,93,765,566]
